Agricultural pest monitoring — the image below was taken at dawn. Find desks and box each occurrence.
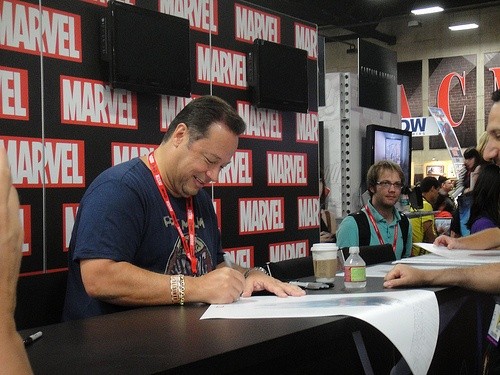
[17,251,500,375]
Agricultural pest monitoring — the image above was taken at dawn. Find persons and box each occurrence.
[0,140,32,375]
[335,161,413,260]
[319,170,337,242]
[59,94,306,326]
[384,88,500,375]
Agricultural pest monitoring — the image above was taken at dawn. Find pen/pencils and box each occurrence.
[223,253,233,268]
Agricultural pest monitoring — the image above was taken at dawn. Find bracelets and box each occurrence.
[180,275,185,305]
[169,275,181,304]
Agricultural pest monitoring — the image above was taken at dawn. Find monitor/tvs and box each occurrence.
[245,39,308,114]
[362,124,413,194]
[99,0,191,98]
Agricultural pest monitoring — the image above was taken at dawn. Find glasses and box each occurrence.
[374,181,404,189]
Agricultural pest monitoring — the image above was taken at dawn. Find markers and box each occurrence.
[22,330,42,347]
[289,279,332,290]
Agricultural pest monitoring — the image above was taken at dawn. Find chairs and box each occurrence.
[265,256,314,283]
[340,243,396,266]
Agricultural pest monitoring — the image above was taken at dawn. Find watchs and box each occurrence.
[244,266,267,276]
